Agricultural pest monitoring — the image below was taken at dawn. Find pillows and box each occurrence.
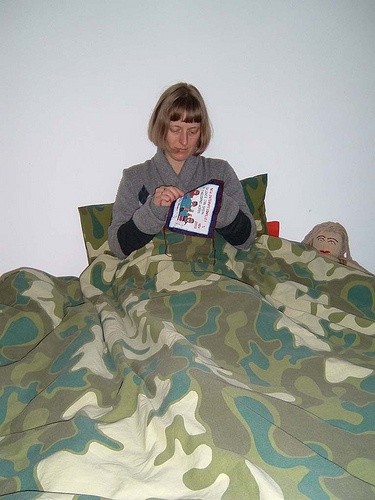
[78,173,268,266]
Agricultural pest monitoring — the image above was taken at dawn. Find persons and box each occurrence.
[107,82,257,259]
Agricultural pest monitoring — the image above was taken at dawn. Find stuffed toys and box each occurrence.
[302,221,352,262]
